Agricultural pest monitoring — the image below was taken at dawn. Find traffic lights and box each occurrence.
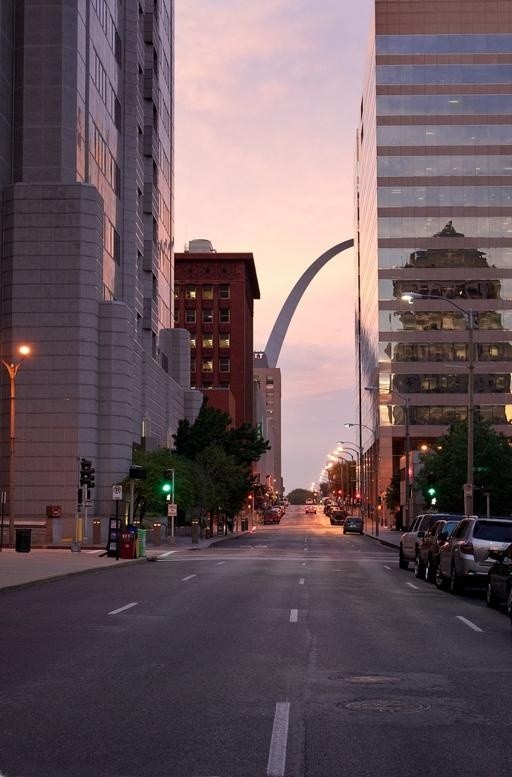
[163,469,172,493]
[427,486,438,510]
[247,493,253,509]
[80,459,95,489]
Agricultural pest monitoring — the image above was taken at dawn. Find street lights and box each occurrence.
[399,292,473,518]
[0,344,33,549]
[311,441,364,517]
[364,386,410,532]
[344,422,379,537]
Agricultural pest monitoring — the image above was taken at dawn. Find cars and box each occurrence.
[305,505,316,514]
[320,497,346,525]
[263,498,289,525]
[343,516,365,535]
[305,498,313,504]
[400,514,512,618]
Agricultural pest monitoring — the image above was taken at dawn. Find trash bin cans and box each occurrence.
[118,530,136,559]
[14,528,32,553]
